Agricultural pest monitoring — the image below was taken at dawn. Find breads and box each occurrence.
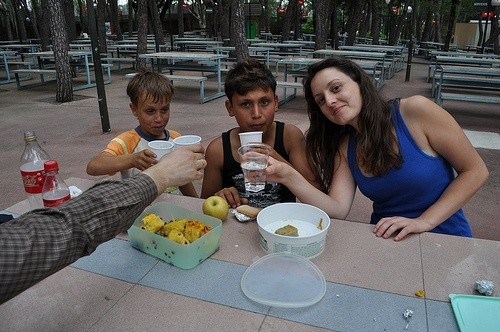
[140,212,213,245]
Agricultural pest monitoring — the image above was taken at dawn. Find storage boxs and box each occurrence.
[115,205,222,266]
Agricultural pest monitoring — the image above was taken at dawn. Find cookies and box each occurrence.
[236,205,260,218]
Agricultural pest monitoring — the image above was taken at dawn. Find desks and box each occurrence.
[214,45,274,71]
[430,50,498,83]
[0,45,42,64]
[175,41,224,52]
[355,44,403,72]
[127,37,155,41]
[314,49,387,87]
[19,51,97,85]
[252,42,306,68]
[275,57,382,99]
[118,40,154,44]
[282,40,316,56]
[174,37,215,42]
[110,43,163,67]
[0,51,18,81]
[0,175,500,332]
[433,55,500,67]
[338,45,397,78]
[437,65,500,102]
[132,34,154,37]
[138,51,229,95]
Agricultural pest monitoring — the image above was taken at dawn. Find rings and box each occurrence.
[201,159,206,168]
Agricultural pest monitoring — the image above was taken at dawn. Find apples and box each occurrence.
[202,196,228,220]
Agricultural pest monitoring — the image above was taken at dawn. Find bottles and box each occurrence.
[41,161,71,208]
[19,131,53,209]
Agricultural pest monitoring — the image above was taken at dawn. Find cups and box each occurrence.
[148,140,174,165]
[237,143,272,193]
[173,135,202,149]
[238,131,263,150]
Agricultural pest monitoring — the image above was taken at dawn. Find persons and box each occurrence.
[200,58,320,208]
[0,145,207,306]
[241,56,490,241]
[79,30,88,50]
[86,66,200,198]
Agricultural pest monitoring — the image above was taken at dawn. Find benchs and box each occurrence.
[69,44,92,48]
[0,47,500,108]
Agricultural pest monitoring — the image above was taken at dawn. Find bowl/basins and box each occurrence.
[128,201,223,269]
[256,202,330,259]
